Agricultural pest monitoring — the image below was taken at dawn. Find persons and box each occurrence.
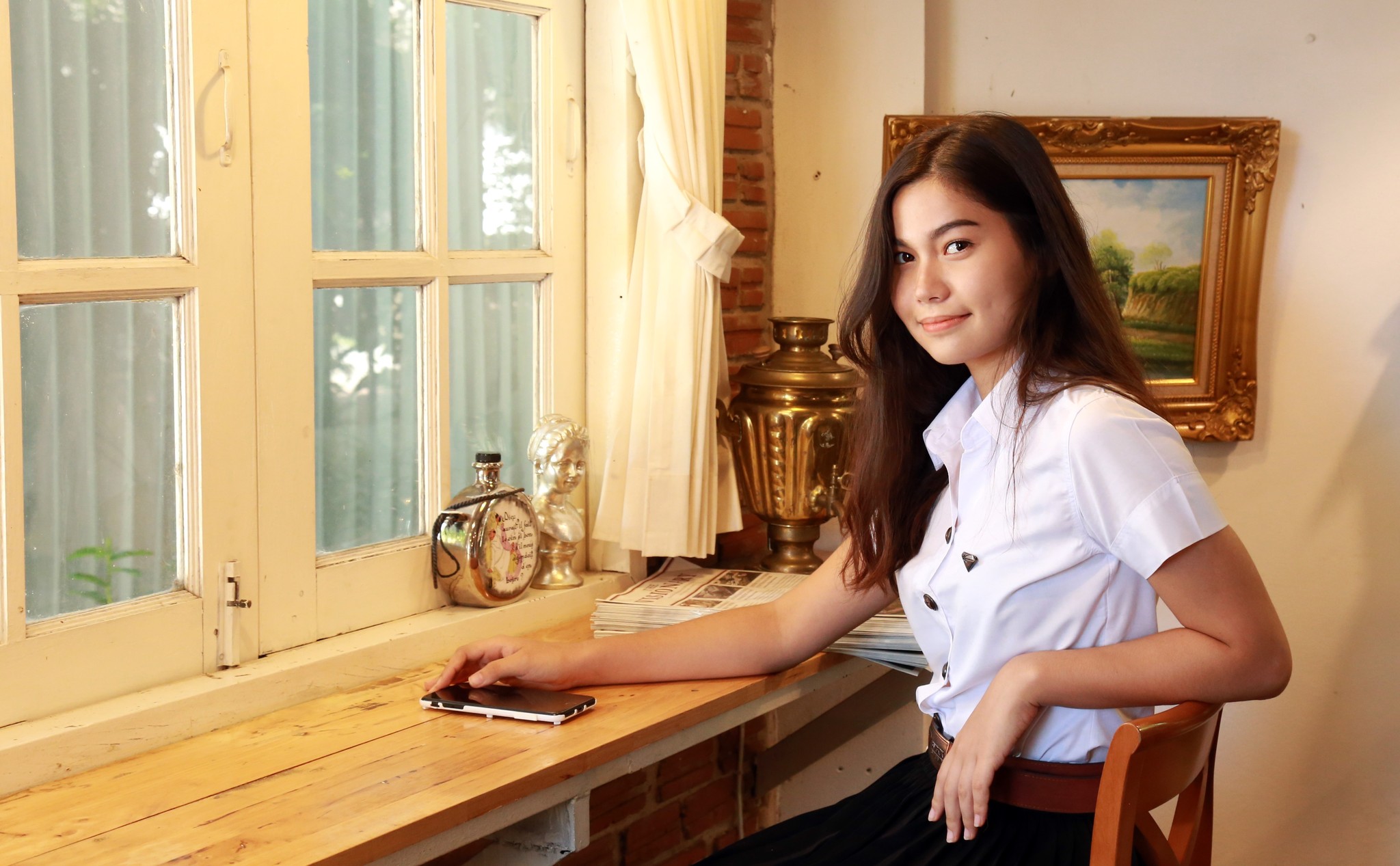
[421,112,1292,866]
[528,413,591,589]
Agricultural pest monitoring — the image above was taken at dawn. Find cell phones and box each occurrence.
[420,681,596,724]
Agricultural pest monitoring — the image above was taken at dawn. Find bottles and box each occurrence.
[437,451,542,608]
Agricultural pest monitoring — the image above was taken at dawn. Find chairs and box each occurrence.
[1088,696,1226,866]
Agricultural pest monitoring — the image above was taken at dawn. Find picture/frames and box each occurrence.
[884,113,1279,446]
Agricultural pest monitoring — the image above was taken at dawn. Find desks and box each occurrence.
[3,601,912,866]
